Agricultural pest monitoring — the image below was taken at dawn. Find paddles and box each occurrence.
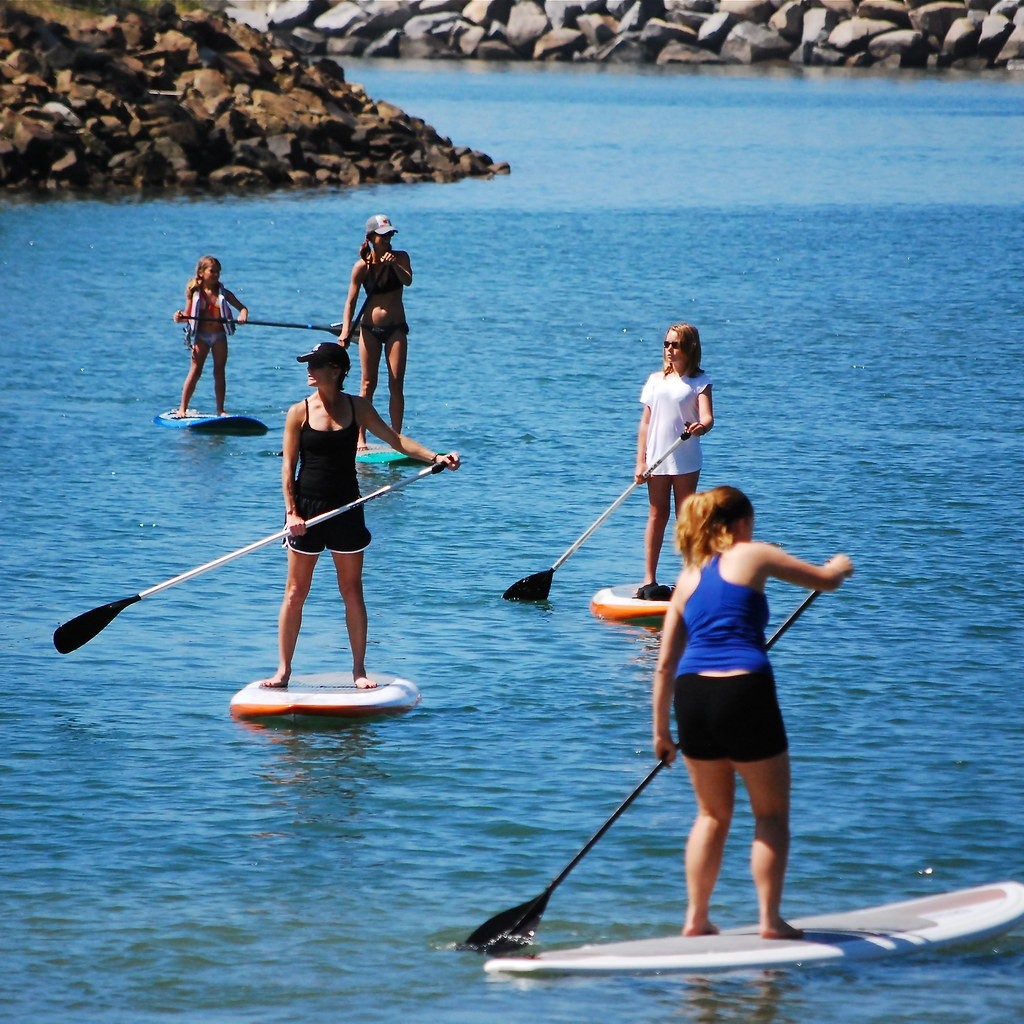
[275,261,389,457]
[457,584,826,955]
[177,311,363,345]
[52,458,448,657]
[501,419,693,602]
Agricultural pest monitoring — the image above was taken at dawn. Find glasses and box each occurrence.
[374,232,394,239]
[308,361,334,368]
[663,341,681,349]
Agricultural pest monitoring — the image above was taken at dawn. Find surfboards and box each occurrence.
[151,405,270,435]
[480,876,1024,979]
[227,666,422,725]
[354,442,414,465]
[587,582,678,625]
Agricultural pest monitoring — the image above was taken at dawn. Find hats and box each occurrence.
[297,341,350,377]
[366,215,398,237]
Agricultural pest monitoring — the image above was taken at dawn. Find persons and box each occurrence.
[652,484,855,940]
[172,256,249,417]
[338,214,412,450]
[260,342,461,690]
[632,322,714,597]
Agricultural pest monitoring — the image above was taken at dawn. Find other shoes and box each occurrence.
[637,583,671,600]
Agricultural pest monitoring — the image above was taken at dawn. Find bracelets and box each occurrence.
[431,452,447,463]
[699,424,706,435]
